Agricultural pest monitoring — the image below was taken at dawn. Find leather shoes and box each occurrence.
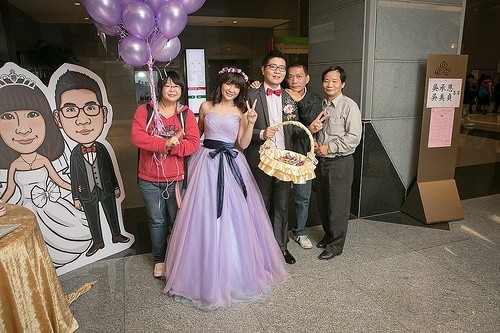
[318,251,334,259]
[317,240,326,248]
[282,249,296,264]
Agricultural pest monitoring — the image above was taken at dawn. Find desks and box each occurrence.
[0,203,79,333]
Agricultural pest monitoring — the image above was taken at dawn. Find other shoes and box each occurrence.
[154,262,164,277]
[293,234,312,248]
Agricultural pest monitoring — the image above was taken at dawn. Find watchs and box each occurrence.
[328,149,331,154]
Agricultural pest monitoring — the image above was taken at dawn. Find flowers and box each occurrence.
[218,67,248,82]
[284,103,296,120]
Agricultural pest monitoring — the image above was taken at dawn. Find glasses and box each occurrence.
[164,83,182,90]
[266,64,287,72]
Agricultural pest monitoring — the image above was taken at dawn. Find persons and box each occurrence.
[463,73,500,114]
[243,50,331,264]
[162,65,292,311]
[250,61,322,250]
[131,70,200,277]
[313,65,362,260]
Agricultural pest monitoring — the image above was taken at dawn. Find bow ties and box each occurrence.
[267,88,281,96]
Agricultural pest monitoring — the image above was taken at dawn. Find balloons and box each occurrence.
[83,0,206,68]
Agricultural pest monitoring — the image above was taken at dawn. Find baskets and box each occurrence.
[258,122,317,184]
